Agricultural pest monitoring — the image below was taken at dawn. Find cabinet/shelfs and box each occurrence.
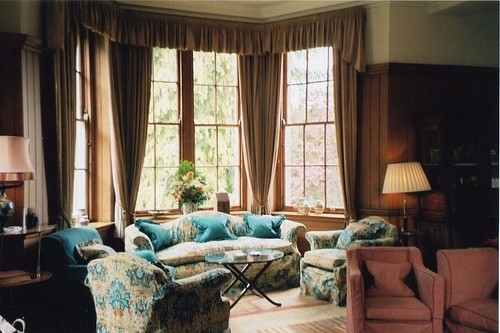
[417,103,498,263]
[0,224,58,306]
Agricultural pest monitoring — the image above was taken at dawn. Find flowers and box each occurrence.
[169,161,212,204]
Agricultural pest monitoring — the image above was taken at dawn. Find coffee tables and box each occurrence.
[203,250,283,310]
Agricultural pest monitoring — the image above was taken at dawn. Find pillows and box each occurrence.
[192,215,237,242]
[365,260,415,297]
[75,240,116,258]
[242,212,285,237]
[334,219,386,248]
[135,219,173,250]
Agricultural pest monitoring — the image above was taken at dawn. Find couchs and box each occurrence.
[345,246,444,333]
[39,227,155,293]
[437,247,498,333]
[85,255,231,333]
[300,216,399,305]
[124,210,306,292]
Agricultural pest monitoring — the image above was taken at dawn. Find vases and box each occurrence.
[183,202,198,217]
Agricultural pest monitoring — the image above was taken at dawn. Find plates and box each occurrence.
[3,226,22,234]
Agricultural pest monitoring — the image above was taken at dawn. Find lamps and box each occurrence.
[0,136,35,230]
[382,162,431,232]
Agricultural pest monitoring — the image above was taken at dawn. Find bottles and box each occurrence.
[297,197,310,215]
[77,208,89,227]
[70,214,76,226]
[26,207,38,227]
[314,200,325,214]
